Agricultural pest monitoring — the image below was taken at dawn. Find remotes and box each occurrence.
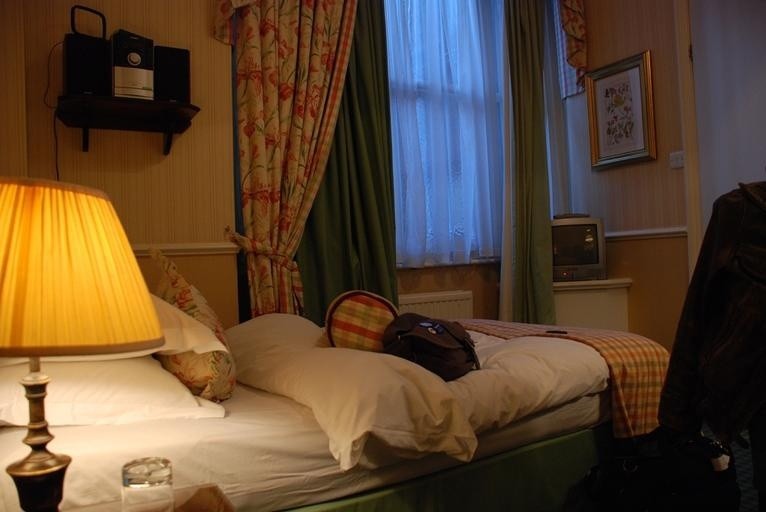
[553,213,590,218]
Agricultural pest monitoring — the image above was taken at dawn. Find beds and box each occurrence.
[0,317,669,509]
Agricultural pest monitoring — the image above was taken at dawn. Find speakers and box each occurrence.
[62,33,112,97]
[154,45,190,104]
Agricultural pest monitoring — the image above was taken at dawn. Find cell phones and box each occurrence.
[546,331,568,334]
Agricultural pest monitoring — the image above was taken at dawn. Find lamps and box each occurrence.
[0,177,167,510]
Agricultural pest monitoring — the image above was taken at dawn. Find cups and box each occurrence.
[121,457,173,509]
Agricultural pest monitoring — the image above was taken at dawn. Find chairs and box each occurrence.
[571,188,766,508]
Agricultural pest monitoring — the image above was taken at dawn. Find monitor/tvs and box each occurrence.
[551,217,607,282]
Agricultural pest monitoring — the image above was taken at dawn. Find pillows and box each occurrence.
[0,292,230,365]
[1,357,227,428]
[150,252,237,402]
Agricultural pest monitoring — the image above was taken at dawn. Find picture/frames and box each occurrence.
[583,46,657,173]
